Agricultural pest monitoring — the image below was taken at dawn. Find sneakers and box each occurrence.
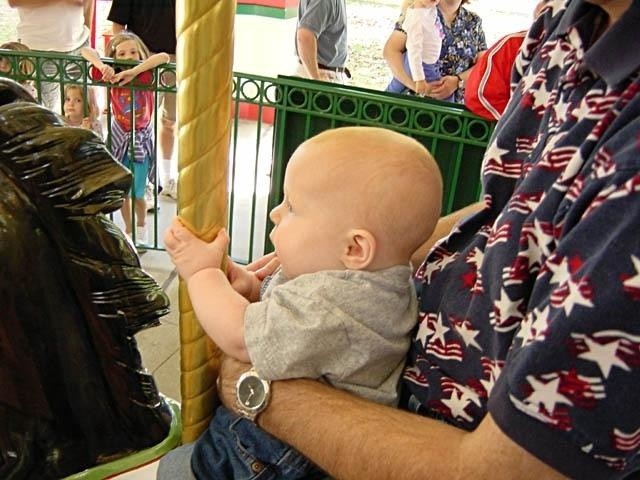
[160,178,177,201]
[136,223,149,253]
[145,181,160,213]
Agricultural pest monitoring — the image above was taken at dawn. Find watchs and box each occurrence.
[233,364,274,426]
[455,74,464,91]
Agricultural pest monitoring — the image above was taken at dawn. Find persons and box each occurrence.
[384,0,455,106]
[59,84,105,144]
[384,0,488,107]
[0,42,39,102]
[107,0,176,211]
[294,0,350,86]
[463,0,548,123]
[80,31,171,245]
[217,0,639,479]
[154,126,444,478]
[9,0,95,116]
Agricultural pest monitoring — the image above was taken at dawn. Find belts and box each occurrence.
[299,58,352,80]
